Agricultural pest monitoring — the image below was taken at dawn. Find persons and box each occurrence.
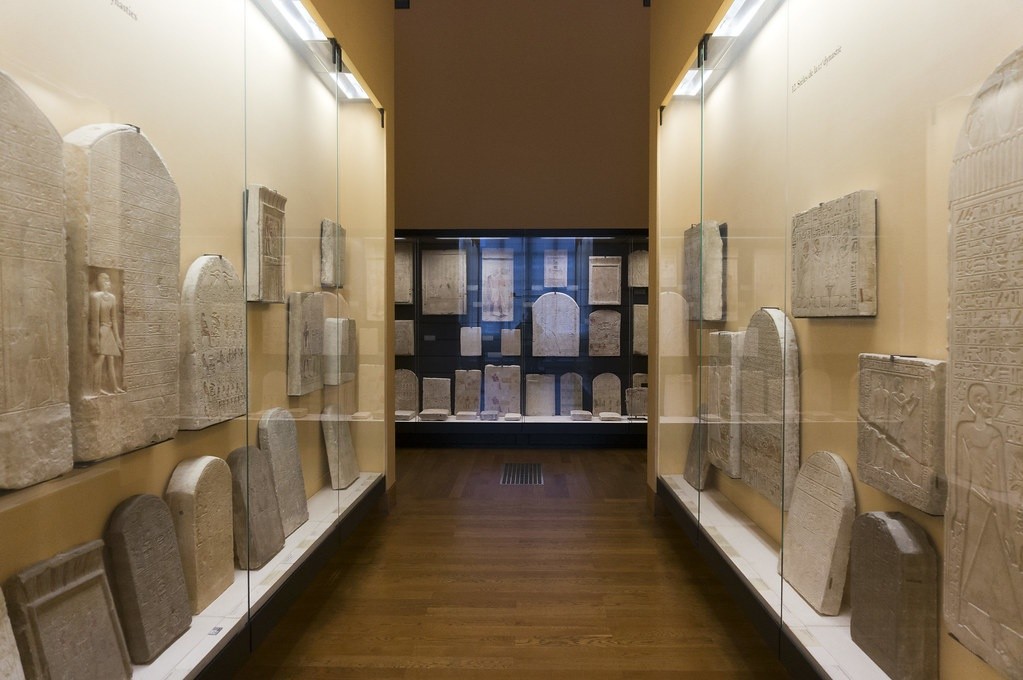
[90,273,122,396]
